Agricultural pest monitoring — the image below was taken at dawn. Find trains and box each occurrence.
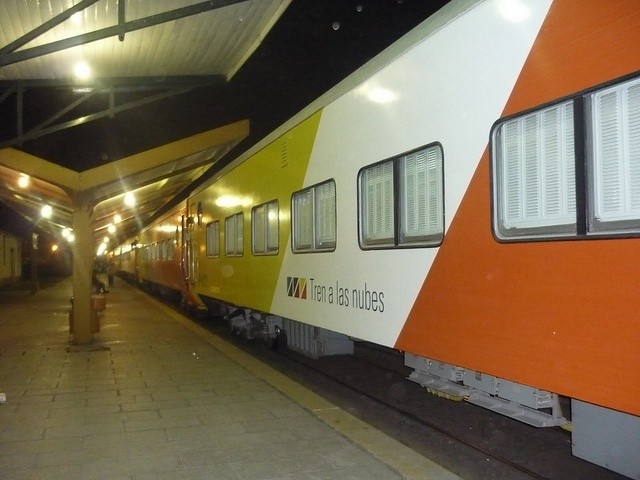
[102,0,640,480]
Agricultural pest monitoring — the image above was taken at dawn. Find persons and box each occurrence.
[107,263,115,286]
[92,269,109,293]
